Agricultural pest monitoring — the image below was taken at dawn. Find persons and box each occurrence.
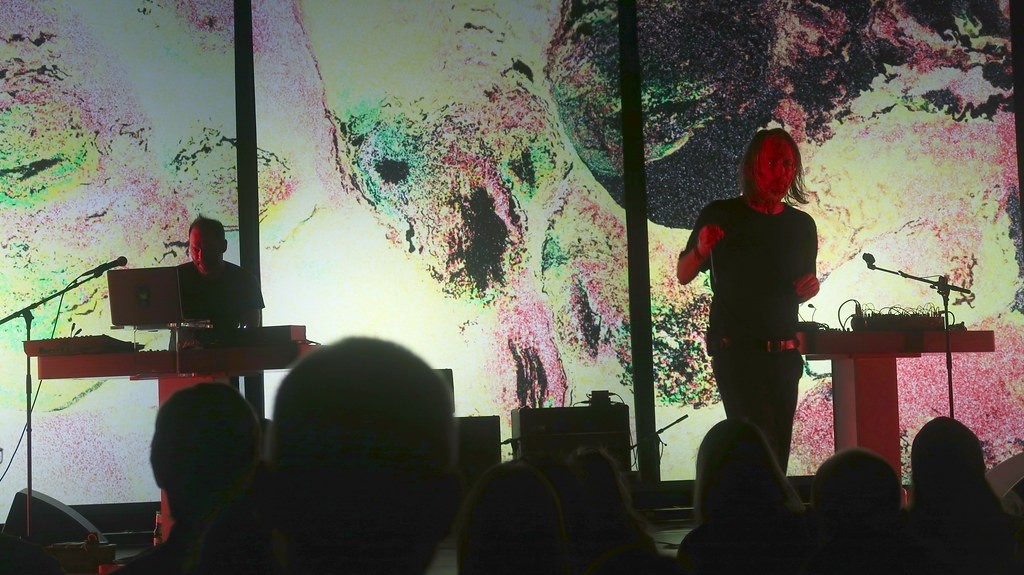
[83,338,1024,575]
[677,127,819,478]
[167,213,262,351]
[675,415,813,575]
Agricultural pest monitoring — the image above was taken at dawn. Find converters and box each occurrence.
[588,390,610,406]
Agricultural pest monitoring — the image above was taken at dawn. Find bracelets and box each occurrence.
[692,244,704,262]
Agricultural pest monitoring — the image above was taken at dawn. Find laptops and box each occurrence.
[106,267,201,325]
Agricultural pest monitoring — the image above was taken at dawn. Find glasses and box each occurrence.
[757,159,798,172]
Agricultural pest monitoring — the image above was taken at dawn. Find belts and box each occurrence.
[749,340,798,355]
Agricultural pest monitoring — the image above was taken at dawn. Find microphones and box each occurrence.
[81,256,127,276]
[808,304,816,322]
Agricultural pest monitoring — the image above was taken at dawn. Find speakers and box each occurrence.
[511,407,631,471]
[984,451,1024,518]
[0,488,108,548]
[452,415,501,470]
